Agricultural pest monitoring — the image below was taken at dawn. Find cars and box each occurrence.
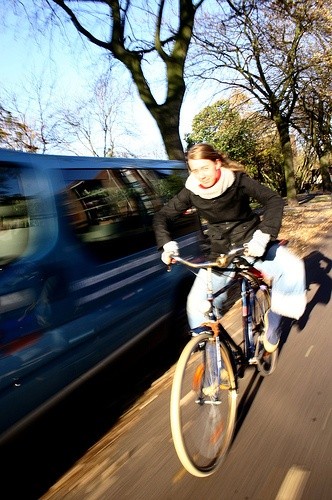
[0,149,195,443]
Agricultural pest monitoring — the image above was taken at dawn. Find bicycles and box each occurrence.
[167,239,289,480]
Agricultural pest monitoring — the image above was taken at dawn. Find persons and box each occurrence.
[152,143,306,396]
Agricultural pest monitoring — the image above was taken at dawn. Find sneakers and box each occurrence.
[265,309,283,352]
